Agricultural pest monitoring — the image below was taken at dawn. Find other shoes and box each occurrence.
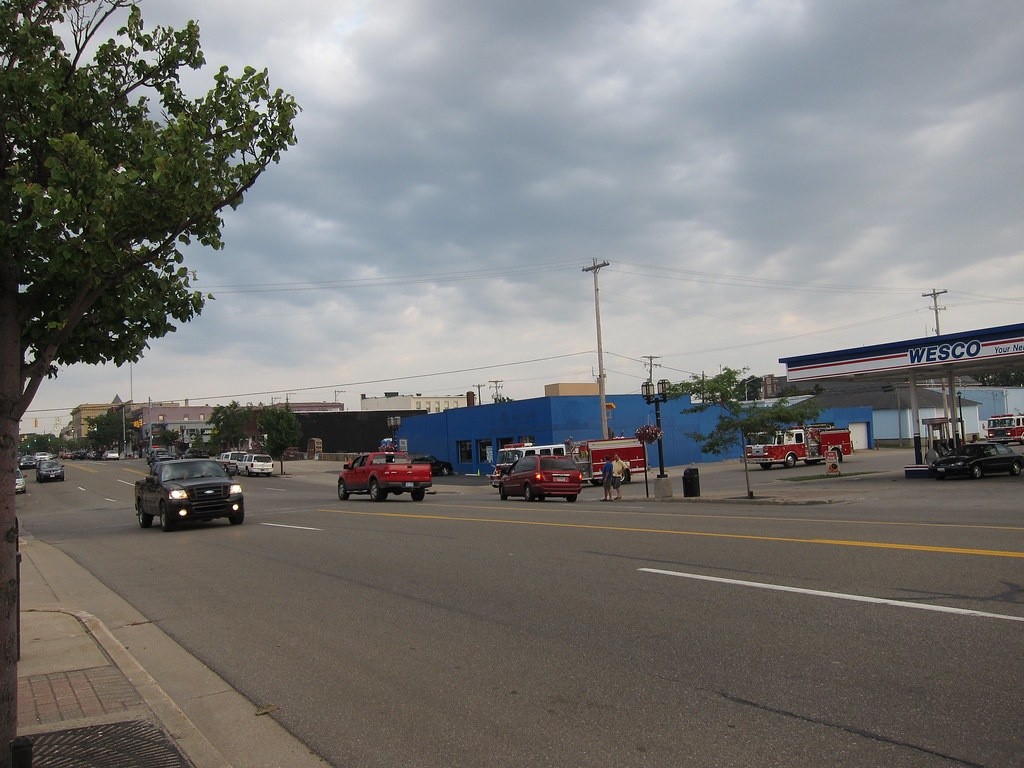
[599,499,608,501]
[614,496,622,501]
[607,499,613,502]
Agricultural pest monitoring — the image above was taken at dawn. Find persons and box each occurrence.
[611,453,627,501]
[599,455,613,502]
[970,434,978,443]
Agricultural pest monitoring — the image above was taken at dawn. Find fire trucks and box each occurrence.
[982,414,1024,446]
[745,422,859,470]
[489,437,648,488]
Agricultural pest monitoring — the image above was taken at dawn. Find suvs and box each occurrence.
[235,454,274,478]
[216,451,248,472]
[498,454,582,503]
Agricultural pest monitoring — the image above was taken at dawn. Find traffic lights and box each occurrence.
[139,419,142,427]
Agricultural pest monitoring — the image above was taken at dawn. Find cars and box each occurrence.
[145,447,176,467]
[929,441,1024,481]
[35,460,65,483]
[102,449,119,461]
[179,447,210,459]
[411,454,454,477]
[14,448,105,464]
[19,455,37,470]
[15,467,28,494]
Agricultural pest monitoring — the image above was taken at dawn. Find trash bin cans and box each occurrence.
[682,467,700,497]
[972,434,977,441]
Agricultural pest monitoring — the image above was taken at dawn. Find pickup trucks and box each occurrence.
[134,459,244,532]
[337,450,433,502]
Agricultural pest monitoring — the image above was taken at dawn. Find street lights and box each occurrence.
[641,378,671,479]
